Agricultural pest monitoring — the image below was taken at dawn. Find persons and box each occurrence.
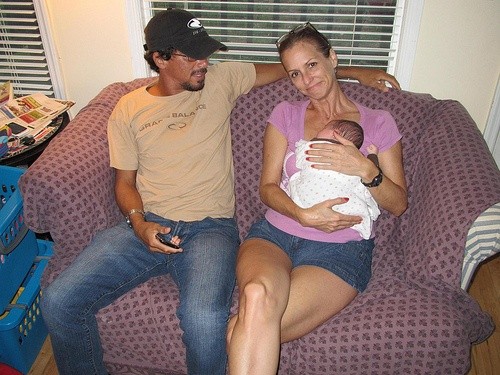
[39,8,401,375]
[228,22,407,375]
[282,119,380,240]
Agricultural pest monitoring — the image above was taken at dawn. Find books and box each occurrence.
[0,80,75,139]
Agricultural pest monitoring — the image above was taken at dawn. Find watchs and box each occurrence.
[125,209,146,228]
[361,168,383,188]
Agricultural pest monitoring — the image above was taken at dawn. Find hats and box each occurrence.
[144,7,228,60]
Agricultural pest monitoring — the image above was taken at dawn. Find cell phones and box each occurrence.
[156,234,179,249]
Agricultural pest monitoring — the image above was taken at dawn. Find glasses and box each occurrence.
[276,22,331,57]
[157,50,199,62]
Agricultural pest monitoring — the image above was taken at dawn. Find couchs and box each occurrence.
[18,78,500,375]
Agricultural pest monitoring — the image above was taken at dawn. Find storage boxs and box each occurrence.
[0,164,60,374]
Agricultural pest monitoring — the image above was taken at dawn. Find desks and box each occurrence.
[0,93,71,167]
